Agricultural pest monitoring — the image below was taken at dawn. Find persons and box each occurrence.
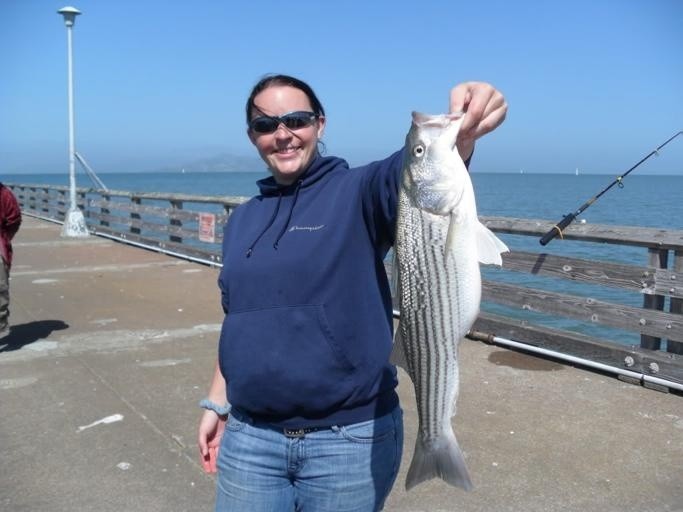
[198,76,509,512]
[0,182,21,352]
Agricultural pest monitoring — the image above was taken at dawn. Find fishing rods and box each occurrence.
[539,132,683,245]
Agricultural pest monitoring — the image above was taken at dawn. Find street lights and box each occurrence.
[57,7,82,209]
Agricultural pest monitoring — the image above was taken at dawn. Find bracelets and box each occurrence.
[199,399,232,416]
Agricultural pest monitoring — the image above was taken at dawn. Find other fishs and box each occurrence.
[387,109,512,494]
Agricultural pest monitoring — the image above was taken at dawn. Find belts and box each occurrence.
[281,425,316,438]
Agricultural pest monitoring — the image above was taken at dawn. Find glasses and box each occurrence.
[249,111,321,133]
